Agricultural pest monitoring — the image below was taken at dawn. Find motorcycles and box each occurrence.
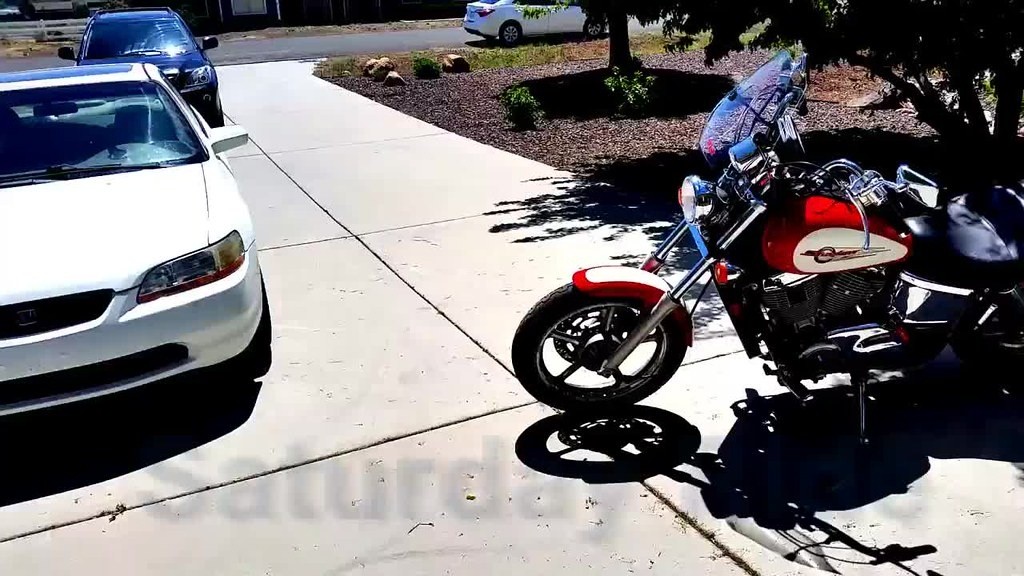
[512,45,1024,449]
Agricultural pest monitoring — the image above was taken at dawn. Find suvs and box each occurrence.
[58,6,224,129]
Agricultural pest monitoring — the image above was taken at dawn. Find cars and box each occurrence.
[1,61,272,433]
[463,0,608,49]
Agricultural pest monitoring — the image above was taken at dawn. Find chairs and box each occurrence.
[110,104,166,151]
